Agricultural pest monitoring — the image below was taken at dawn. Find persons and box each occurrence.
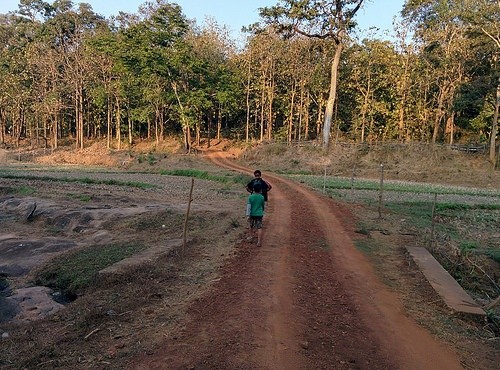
[245,170,272,227]
[246,184,265,247]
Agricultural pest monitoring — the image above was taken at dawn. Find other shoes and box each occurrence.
[256,243,262,247]
[247,237,253,242]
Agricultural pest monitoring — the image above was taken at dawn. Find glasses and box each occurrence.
[255,175,260,177]
